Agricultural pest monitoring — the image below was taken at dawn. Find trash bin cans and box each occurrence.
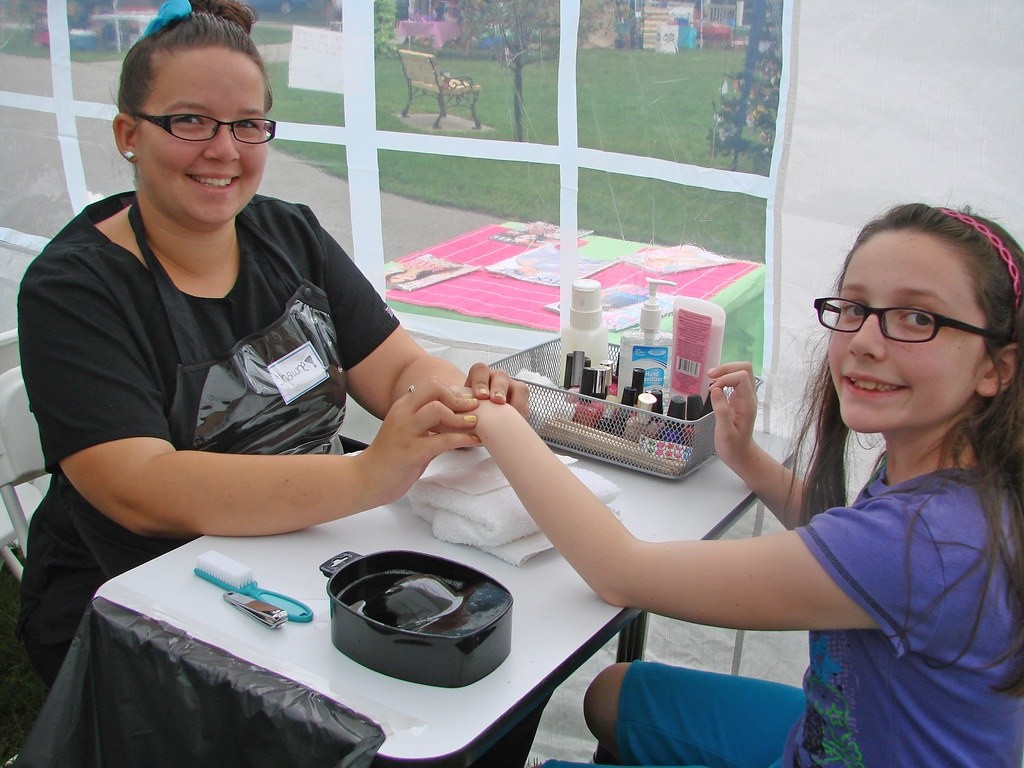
[8,596,388,768]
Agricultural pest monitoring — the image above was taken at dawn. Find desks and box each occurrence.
[398,19,461,47]
[92,428,794,768]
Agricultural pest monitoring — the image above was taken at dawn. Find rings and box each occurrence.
[408,384,415,392]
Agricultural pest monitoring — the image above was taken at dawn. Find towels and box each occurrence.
[405,455,624,569]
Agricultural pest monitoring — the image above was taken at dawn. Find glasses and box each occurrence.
[814,297,1012,349]
[133,113,276,144]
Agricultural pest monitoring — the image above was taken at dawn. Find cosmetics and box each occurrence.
[559,277,726,447]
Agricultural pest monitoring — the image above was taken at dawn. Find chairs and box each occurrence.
[0,328,52,585]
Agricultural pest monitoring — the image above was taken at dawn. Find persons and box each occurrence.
[423,204,1024,768]
[13,1,529,691]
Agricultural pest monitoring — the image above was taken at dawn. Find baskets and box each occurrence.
[479,331,763,481]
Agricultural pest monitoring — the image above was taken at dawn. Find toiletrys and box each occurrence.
[560,277,609,389]
[617,276,678,404]
[668,294,727,419]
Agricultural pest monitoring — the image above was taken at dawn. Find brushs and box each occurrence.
[194,550,314,622]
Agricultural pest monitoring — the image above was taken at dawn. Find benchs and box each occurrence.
[397,49,483,130]
